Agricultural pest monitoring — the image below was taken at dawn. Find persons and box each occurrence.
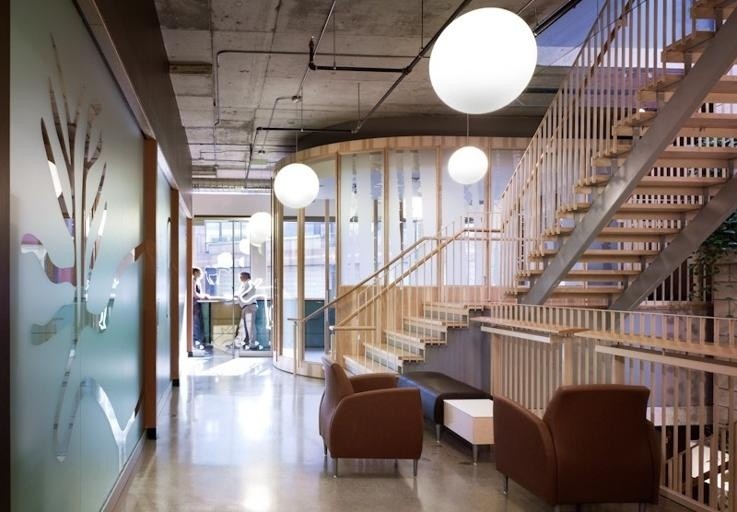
[222,271,258,350]
[191,266,212,351]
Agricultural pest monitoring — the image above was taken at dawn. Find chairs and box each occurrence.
[494,384,661,512]
[318,355,424,478]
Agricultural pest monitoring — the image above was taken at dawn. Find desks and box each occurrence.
[197,299,272,351]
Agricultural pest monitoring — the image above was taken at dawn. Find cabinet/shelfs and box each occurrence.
[304,298,335,351]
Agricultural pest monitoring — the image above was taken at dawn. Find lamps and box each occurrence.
[429,5,538,115]
[437,116,495,186]
[273,95,320,211]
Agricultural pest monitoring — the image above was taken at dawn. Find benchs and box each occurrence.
[396,372,494,467]
